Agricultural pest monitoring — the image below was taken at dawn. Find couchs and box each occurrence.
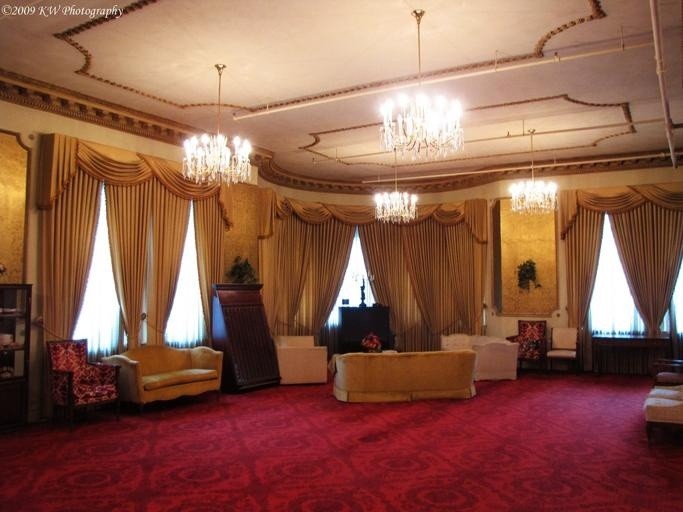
[331,350,477,403]
[103,343,224,410]
[437,332,518,380]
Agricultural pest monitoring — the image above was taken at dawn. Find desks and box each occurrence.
[591,334,672,377]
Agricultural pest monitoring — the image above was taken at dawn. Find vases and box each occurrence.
[365,349,380,352]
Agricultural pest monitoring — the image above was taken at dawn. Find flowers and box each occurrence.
[360,334,381,349]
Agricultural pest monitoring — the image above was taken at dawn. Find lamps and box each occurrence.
[370,143,421,227]
[508,123,559,218]
[177,52,252,192]
[378,2,470,161]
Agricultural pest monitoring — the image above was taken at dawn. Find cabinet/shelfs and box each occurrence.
[337,305,393,342]
[0,283,33,434]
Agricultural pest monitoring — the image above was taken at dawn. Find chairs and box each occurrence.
[45,337,120,428]
[643,351,683,446]
[504,320,547,370]
[275,334,329,386]
[546,326,580,370]
